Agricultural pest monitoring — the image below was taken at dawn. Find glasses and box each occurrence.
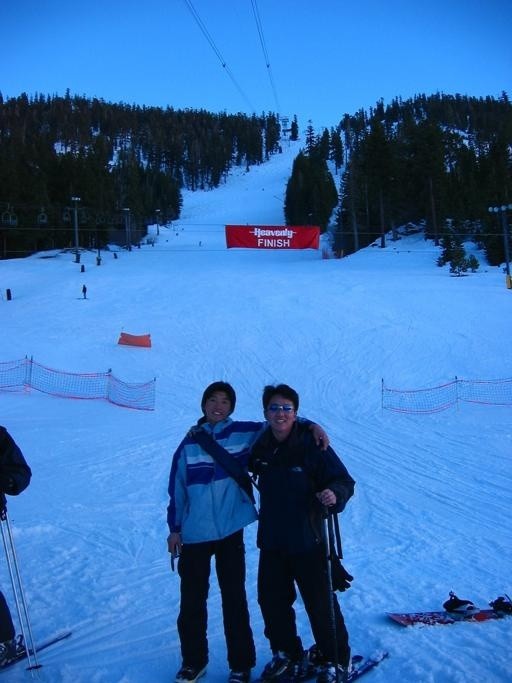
[268,404,293,413]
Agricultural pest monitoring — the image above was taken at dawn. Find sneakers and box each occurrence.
[175,659,349,683]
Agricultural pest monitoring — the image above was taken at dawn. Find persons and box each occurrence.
[239,383,359,681]
[164,379,332,682]
[1,425,35,667]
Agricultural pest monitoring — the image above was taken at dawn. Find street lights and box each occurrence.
[154,208,160,236]
[488,201,511,288]
[124,207,131,247]
[72,193,82,253]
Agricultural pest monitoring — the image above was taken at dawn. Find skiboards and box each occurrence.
[387,609,506,627]
[2,630,70,666]
[347,650,387,683]
